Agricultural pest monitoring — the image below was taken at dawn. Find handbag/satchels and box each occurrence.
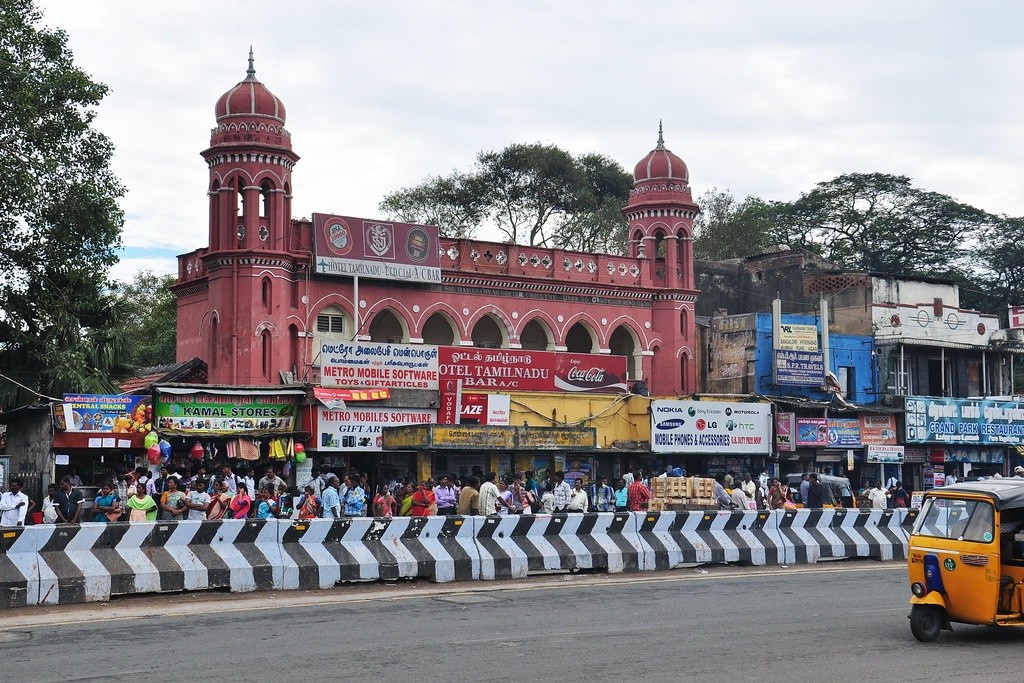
[178,499,185,508]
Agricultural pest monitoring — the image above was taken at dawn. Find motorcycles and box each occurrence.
[907,478,1024,642]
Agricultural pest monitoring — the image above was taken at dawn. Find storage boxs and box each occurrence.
[647,476,718,511]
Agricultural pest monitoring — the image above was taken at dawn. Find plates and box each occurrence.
[72,411,112,430]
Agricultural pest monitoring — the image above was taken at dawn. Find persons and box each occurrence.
[1013,466,1024,477]
[714,467,910,510]
[950,501,992,542]
[0,464,673,527]
[945,469,957,487]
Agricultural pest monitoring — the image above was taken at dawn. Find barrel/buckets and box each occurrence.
[33,512,42,524]
